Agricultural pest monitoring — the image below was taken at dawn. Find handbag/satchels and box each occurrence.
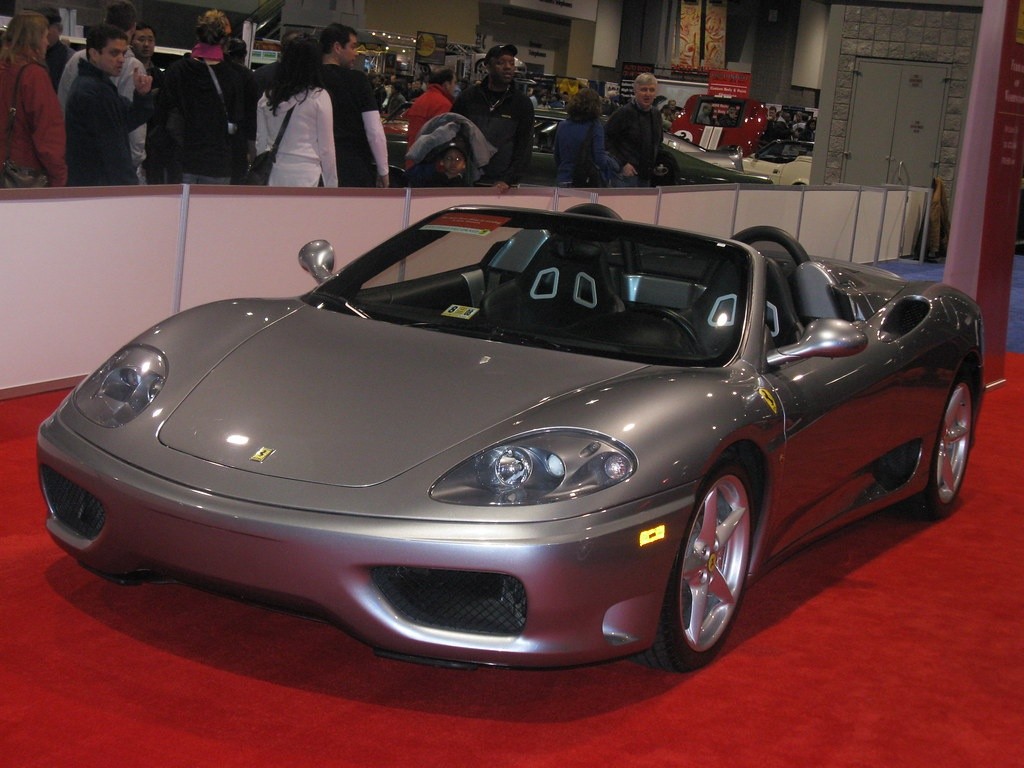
[243,151,276,185]
[1,160,48,188]
[573,120,596,175]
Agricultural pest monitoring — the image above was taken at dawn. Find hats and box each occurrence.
[484,43,517,66]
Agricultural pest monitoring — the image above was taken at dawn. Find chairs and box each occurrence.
[516,203,632,317]
[669,257,799,350]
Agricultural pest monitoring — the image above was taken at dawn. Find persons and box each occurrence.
[0,11,68,189]
[603,73,667,188]
[319,22,389,189]
[367,71,484,116]
[162,10,254,187]
[554,88,612,187]
[528,83,626,117]
[31,2,389,186]
[403,135,474,190]
[65,24,154,188]
[255,36,338,187]
[659,100,741,136]
[405,69,458,175]
[450,44,534,190]
[764,106,816,144]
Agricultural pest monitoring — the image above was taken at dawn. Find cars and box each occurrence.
[378,95,814,187]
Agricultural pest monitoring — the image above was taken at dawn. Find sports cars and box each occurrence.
[36,200,988,674]
